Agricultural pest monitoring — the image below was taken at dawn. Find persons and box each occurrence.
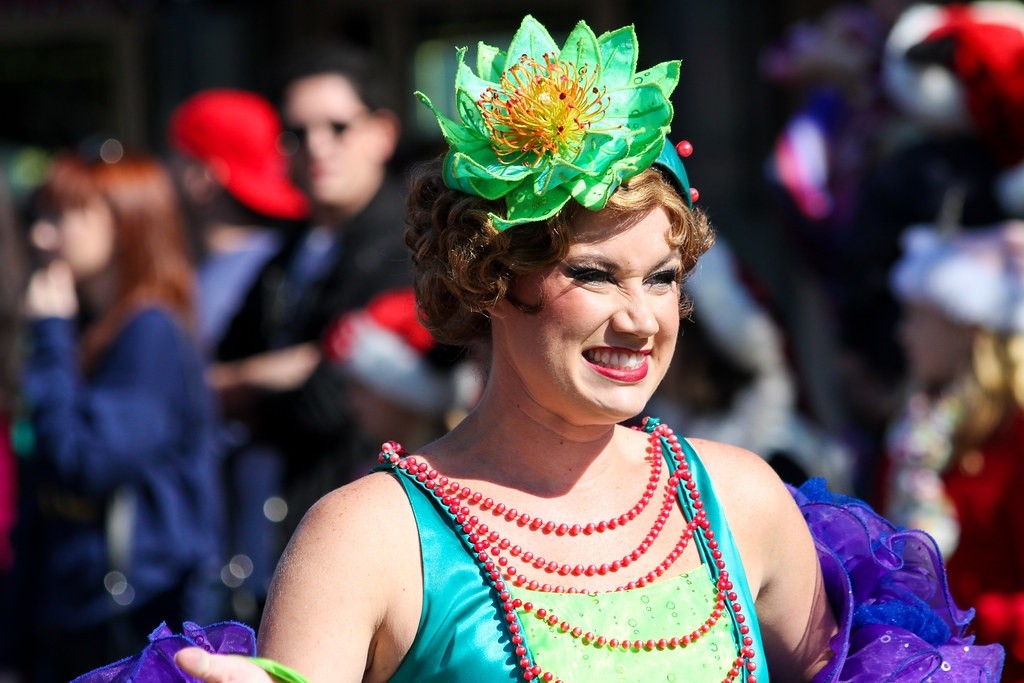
[640,1,1024,683]
[67,14,1005,683]
[0,46,491,683]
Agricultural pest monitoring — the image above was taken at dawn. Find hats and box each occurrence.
[330,290,455,418]
[166,88,313,220]
[886,224,1024,335]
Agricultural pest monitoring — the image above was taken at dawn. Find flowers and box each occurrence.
[413,14,684,232]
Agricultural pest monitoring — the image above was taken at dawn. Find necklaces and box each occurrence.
[378,417,758,683]
